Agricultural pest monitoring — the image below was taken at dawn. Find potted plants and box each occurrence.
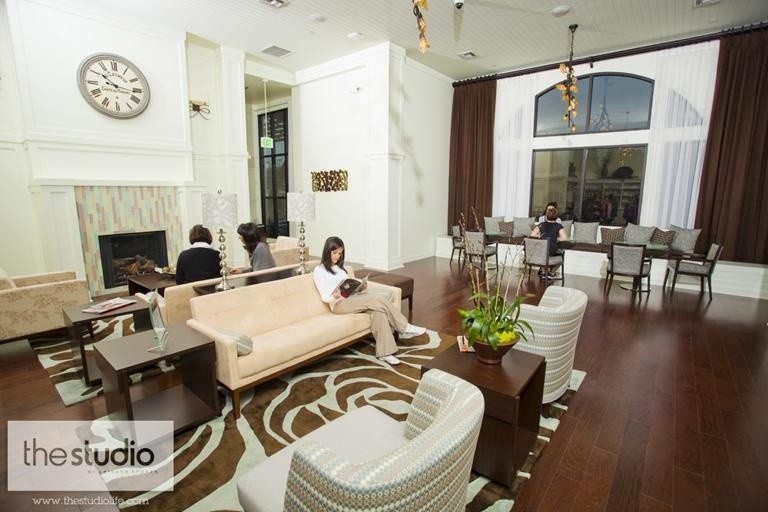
[454,205,545,362]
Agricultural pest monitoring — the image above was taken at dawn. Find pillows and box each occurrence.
[214,327,256,357]
[363,282,395,303]
[482,215,703,255]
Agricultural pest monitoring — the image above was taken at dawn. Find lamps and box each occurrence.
[286,189,315,275]
[200,187,238,290]
[591,76,612,132]
[615,111,633,167]
[555,23,582,133]
[408,0,433,54]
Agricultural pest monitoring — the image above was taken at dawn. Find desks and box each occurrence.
[421,340,546,490]
[93,324,223,452]
[62,293,151,387]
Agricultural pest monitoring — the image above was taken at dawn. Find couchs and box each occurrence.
[147,258,322,351]
[184,265,403,421]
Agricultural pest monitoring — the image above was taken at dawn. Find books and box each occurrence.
[331,271,371,299]
[457,335,475,353]
[80,296,138,315]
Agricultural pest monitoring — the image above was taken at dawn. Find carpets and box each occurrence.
[26,295,183,408]
[75,324,588,512]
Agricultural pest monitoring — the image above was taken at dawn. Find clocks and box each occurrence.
[76,52,150,119]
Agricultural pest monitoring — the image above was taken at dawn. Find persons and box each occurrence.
[312,236,427,365]
[176,224,222,285]
[530,208,568,279]
[228,223,275,274]
[568,191,639,226]
[534,201,561,225]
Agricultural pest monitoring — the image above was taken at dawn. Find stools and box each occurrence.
[354,269,414,323]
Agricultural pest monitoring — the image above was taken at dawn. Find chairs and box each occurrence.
[463,230,499,281]
[238,368,486,512]
[267,234,309,265]
[0,267,89,347]
[602,241,653,302]
[508,282,588,406]
[521,236,565,288]
[662,243,725,301]
[447,225,468,266]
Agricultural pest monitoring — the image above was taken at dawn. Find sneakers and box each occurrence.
[402,324,427,337]
[378,355,400,365]
